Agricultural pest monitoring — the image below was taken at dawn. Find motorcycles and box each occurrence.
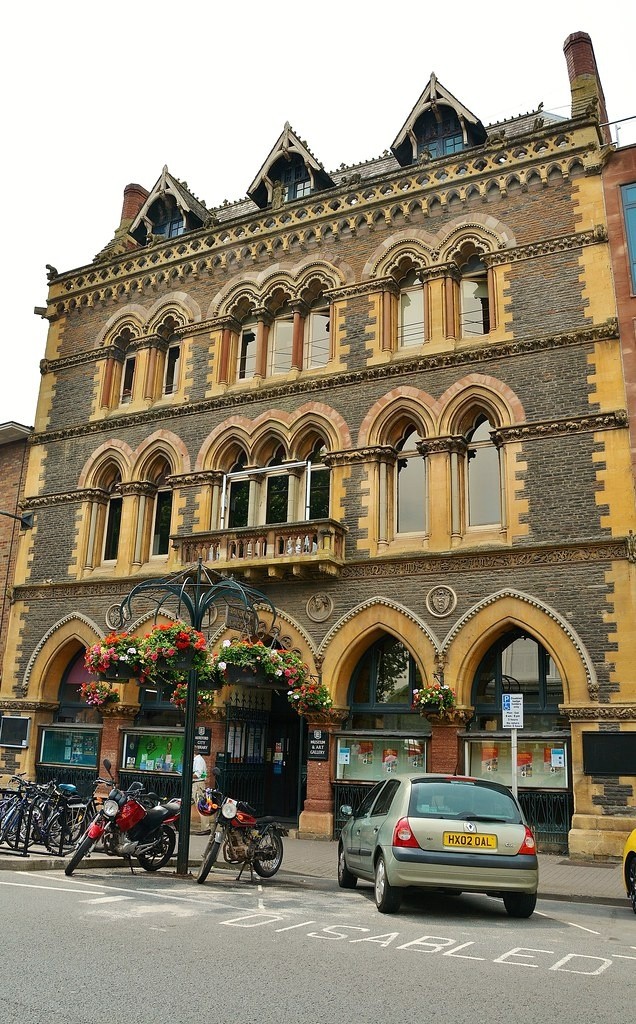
[64,759,194,876]
[194,767,292,884]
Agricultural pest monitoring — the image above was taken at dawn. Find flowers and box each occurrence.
[412,684,457,716]
[83,622,309,687]
[77,683,120,706]
[287,685,333,715]
[169,684,215,713]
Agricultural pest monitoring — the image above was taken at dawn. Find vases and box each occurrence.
[106,661,138,679]
[257,669,292,689]
[228,665,257,683]
[157,656,190,671]
[423,703,440,714]
[97,672,129,683]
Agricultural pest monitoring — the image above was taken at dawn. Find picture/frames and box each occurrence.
[93,700,107,707]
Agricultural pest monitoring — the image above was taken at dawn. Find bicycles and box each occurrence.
[0,771,112,856]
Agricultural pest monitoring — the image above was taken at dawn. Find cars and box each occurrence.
[622,829,636,915]
[336,771,541,920]
